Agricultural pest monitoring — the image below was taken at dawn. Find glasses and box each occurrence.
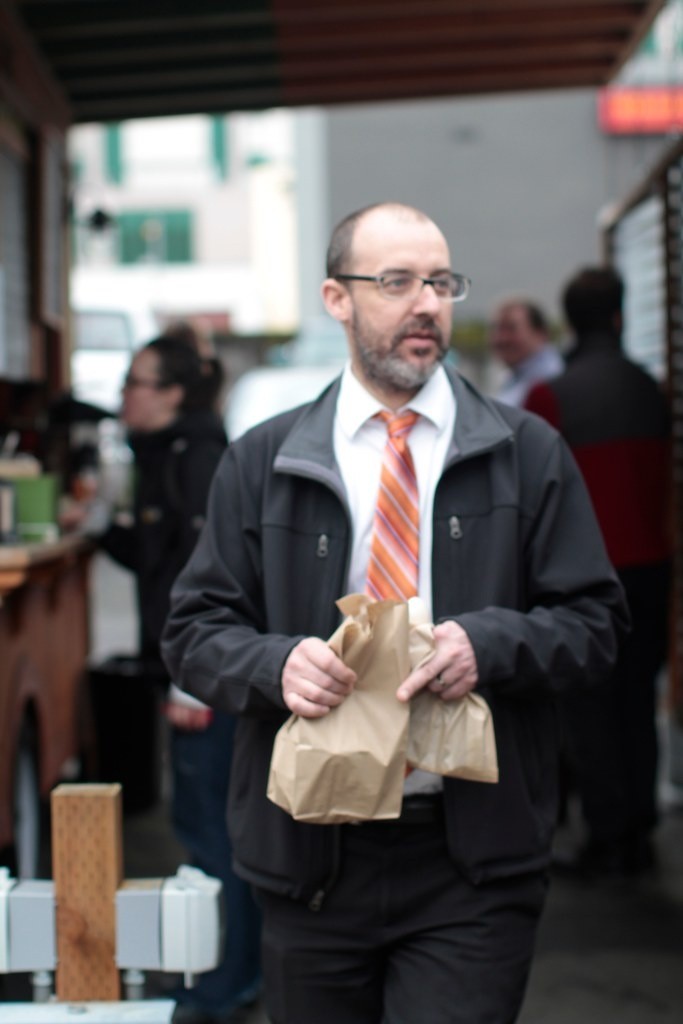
[335,269,472,304]
[124,370,156,392]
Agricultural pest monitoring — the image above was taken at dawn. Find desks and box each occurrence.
[0,533,98,875]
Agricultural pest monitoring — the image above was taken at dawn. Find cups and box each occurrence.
[0,460,59,545]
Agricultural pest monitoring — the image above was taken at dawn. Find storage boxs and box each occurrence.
[0,484,16,545]
[15,473,60,543]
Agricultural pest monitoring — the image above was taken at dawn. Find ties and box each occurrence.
[363,410,420,779]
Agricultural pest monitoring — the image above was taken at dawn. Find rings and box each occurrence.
[437,674,450,689]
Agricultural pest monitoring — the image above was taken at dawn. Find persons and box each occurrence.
[46,263,682,1024]
[160,200,634,1024]
